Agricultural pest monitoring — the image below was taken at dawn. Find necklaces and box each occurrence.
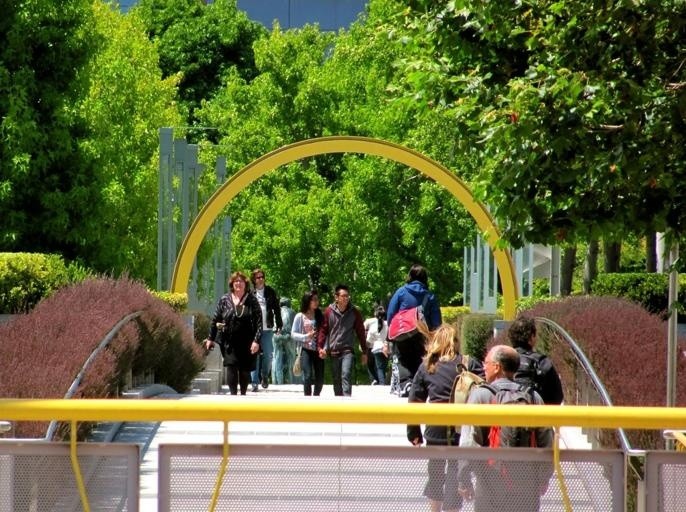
[234,302,245,318]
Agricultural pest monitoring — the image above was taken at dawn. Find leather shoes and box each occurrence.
[251,386,258,392]
[261,374,269,389]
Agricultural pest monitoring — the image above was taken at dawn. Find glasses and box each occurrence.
[337,294,349,298]
[481,360,496,366]
[255,276,264,280]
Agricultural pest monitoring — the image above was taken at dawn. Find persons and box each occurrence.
[316,283,369,397]
[359,305,379,385]
[386,264,443,376]
[507,314,565,405]
[290,289,329,396]
[249,268,284,393]
[272,296,298,385]
[364,306,393,385]
[405,321,486,512]
[203,270,263,396]
[455,343,557,512]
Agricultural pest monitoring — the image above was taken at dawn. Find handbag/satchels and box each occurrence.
[387,306,427,342]
[292,356,304,377]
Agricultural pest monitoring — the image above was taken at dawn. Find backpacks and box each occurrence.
[479,383,538,480]
[516,353,547,390]
[449,354,485,435]
